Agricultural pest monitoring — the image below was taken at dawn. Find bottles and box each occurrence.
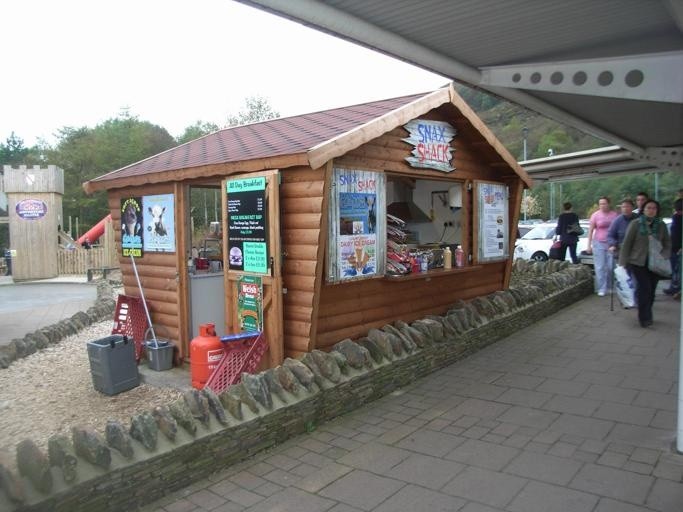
[186,256,193,275]
[454,244,465,268]
[443,246,451,269]
[409,252,429,272]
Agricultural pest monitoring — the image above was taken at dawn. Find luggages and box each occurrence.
[550,228,561,260]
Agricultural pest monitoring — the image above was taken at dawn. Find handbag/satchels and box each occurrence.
[614,264,637,310]
[567,223,584,235]
[647,234,673,278]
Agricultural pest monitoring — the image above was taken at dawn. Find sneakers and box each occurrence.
[664,289,681,299]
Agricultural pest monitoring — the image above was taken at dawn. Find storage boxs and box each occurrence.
[87,333,139,396]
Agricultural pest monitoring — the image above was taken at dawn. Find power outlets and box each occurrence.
[443,220,455,227]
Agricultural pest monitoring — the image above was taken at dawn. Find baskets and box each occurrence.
[112,293,150,361]
[205,332,270,395]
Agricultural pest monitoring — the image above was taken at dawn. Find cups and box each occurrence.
[208,261,222,273]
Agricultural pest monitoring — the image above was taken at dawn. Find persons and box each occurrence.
[662,197,683,303]
[4,246,12,276]
[635,191,651,215]
[551,203,582,265]
[585,195,619,298]
[606,199,639,311]
[617,198,672,329]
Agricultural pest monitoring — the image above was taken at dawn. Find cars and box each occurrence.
[513,217,673,260]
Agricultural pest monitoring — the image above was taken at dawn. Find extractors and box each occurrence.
[386,179,433,224]
